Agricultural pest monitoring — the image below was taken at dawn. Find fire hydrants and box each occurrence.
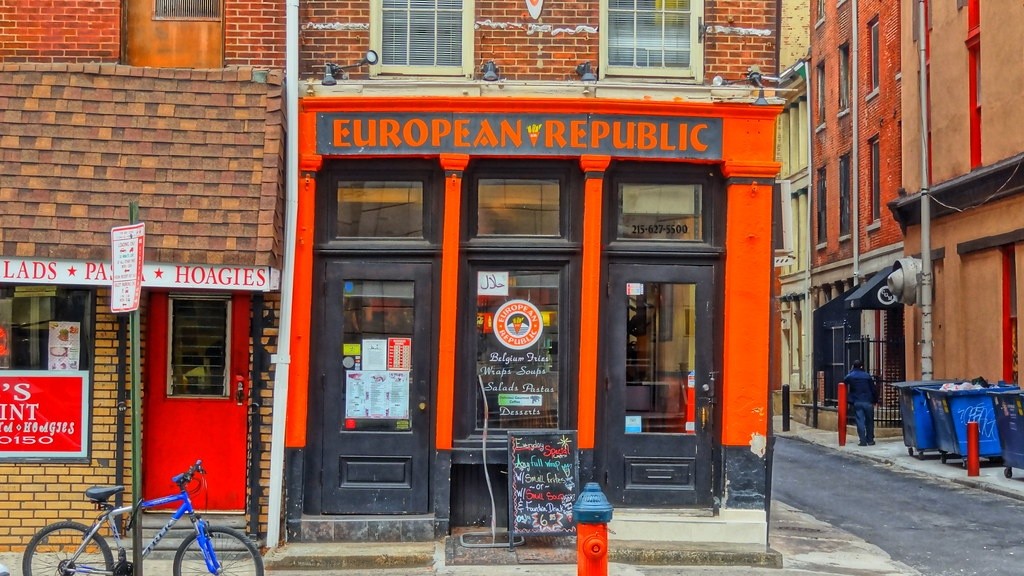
[570,483,614,576]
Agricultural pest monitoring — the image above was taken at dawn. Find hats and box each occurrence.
[854,360,862,368]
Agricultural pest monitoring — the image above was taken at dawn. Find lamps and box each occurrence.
[319,65,335,86]
[483,61,497,80]
[712,75,731,88]
[581,63,596,83]
[356,50,380,66]
[752,91,770,108]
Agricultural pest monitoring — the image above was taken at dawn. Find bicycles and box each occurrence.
[22,458,265,576]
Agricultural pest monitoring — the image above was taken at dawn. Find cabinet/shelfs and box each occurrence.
[535,304,559,371]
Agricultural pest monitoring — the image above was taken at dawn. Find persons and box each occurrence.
[843,360,878,446]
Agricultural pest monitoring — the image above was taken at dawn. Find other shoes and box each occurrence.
[858,441,868,446]
[868,441,875,445]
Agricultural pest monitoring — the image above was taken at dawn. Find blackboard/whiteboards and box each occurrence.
[509,432,577,535]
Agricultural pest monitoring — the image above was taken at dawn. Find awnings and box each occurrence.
[844,265,900,310]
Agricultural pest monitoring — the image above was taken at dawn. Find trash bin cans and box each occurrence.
[888,377,1024,480]
